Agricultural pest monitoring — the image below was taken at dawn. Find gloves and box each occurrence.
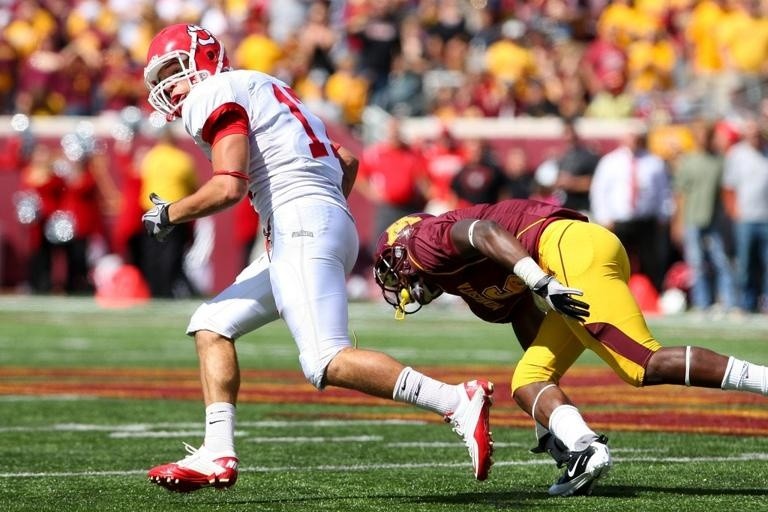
[532,275,590,322]
[529,432,572,468]
[141,193,176,242]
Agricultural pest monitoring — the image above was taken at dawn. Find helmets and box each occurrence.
[374,213,444,313]
[144,24,233,122]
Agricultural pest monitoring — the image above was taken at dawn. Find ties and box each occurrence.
[631,157,641,215]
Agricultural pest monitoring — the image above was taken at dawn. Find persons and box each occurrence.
[0,1,768,320]
[372,196,768,499]
[137,20,497,491]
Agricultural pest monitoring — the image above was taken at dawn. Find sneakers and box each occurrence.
[547,434,609,497]
[147,450,238,492]
[444,379,494,480]
